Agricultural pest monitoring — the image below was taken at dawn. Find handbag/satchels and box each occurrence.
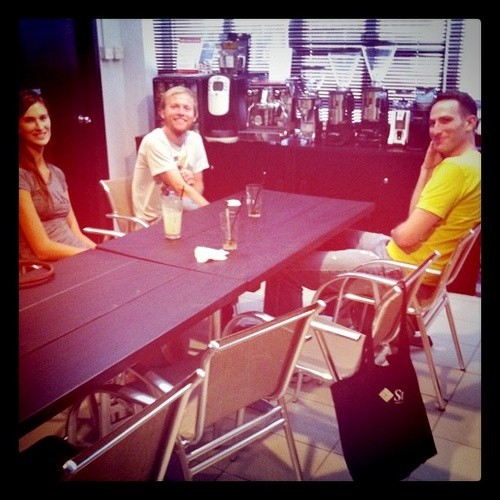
[330,281,437,482]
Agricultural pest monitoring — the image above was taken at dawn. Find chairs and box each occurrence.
[21,179,481,480]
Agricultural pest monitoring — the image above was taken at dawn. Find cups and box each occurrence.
[218,211,238,250]
[160,196,183,240]
[246,183,263,218]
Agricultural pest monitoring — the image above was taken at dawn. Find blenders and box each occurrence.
[324,55,359,145]
[356,46,397,144]
[294,65,325,143]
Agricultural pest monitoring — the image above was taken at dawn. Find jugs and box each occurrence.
[218,41,245,75]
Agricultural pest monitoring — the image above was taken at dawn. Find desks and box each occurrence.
[94,186,368,339]
[18,247,247,439]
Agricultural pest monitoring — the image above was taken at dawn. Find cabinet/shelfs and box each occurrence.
[132,135,482,299]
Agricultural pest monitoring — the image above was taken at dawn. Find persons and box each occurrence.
[264,90,481,317]
[19,88,98,423]
[131,85,234,331]
